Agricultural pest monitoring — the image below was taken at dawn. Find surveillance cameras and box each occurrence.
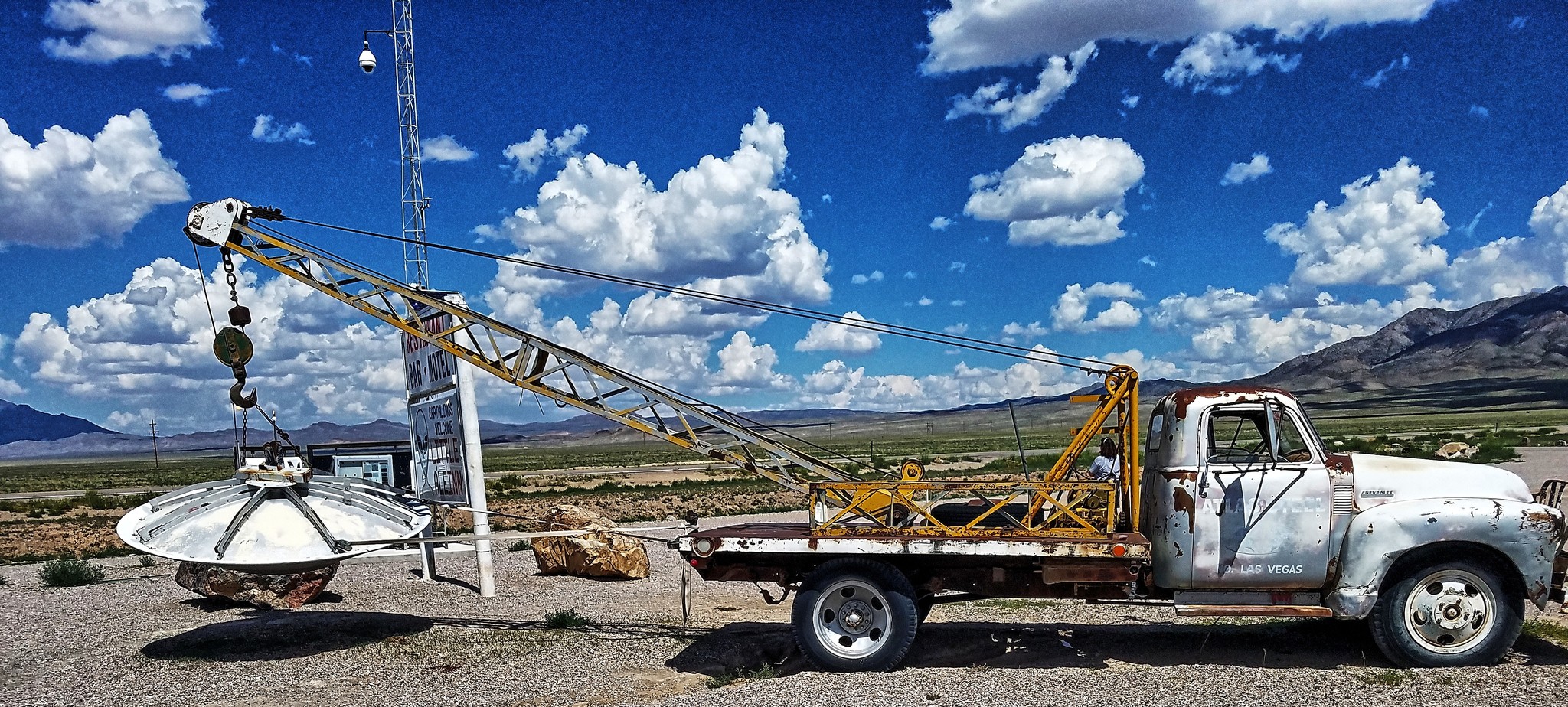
[359,48,376,73]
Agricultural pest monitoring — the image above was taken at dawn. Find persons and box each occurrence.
[1089,437,1130,509]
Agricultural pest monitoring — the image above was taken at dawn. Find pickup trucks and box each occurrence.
[664,366,1568,673]
